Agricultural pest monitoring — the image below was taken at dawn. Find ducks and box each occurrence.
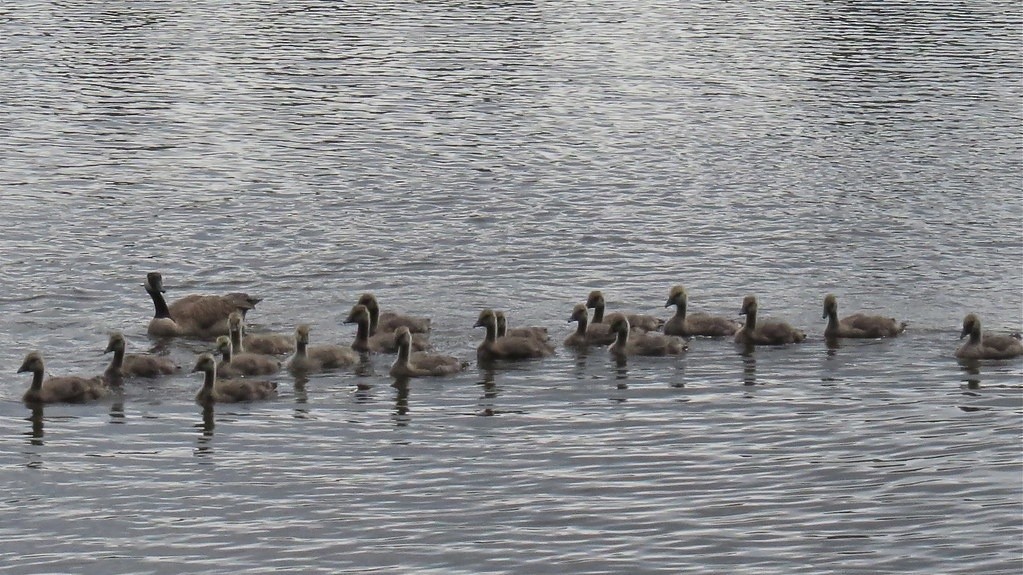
[951,313,1023,359]
[820,295,912,338]
[14,272,809,406]
